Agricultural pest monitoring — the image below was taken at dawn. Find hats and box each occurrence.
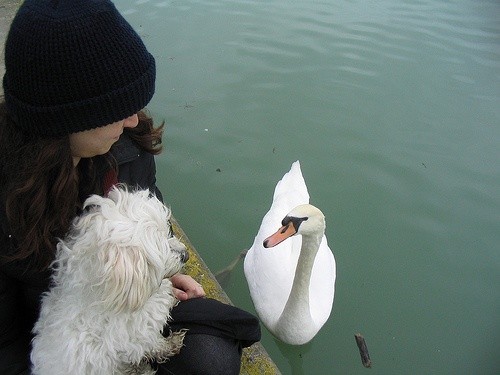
[2,0,158,140]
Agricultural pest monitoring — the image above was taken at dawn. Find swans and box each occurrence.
[212,158,338,348]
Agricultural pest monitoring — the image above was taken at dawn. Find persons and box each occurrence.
[0,2,261,375]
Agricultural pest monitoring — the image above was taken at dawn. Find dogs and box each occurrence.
[31,183,192,375]
